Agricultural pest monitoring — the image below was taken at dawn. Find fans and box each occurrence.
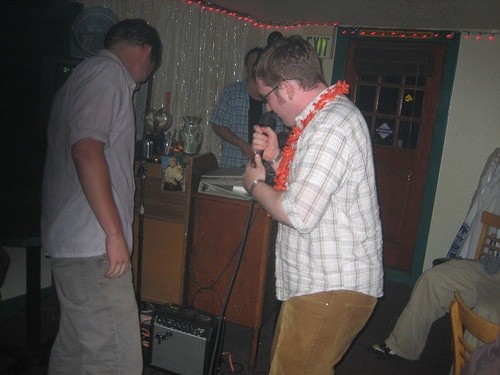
[71,6,119,57]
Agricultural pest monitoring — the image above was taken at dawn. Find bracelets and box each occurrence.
[268,149,282,165]
[249,180,263,197]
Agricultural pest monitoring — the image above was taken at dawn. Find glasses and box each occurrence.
[260,79,287,105]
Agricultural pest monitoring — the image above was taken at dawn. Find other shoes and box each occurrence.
[369,343,397,356]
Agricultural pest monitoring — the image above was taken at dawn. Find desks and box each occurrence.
[185,190,278,375]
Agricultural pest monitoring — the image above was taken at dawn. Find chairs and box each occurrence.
[473,210,500,260]
[449,290,500,375]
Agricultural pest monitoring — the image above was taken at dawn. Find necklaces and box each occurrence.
[275,80,350,191]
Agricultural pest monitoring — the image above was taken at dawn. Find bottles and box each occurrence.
[180,115,203,155]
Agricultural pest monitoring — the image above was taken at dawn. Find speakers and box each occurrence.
[148,302,222,375]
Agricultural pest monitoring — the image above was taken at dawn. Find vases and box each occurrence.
[178,116,204,157]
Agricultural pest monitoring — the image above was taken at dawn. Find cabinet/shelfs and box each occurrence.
[133,151,220,307]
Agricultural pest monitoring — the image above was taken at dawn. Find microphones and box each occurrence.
[250,113,276,161]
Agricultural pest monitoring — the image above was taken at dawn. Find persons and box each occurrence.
[369,259,500,375]
[209,47,290,168]
[41,19,164,375]
[243,34,384,375]
